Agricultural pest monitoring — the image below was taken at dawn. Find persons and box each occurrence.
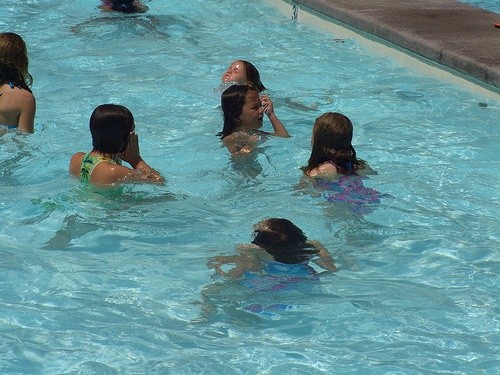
[251,218,307,246]
[214,85,288,135]
[0,32,36,134]
[69,104,142,184]
[222,59,264,91]
[301,112,365,176]
[97,0,149,14]
